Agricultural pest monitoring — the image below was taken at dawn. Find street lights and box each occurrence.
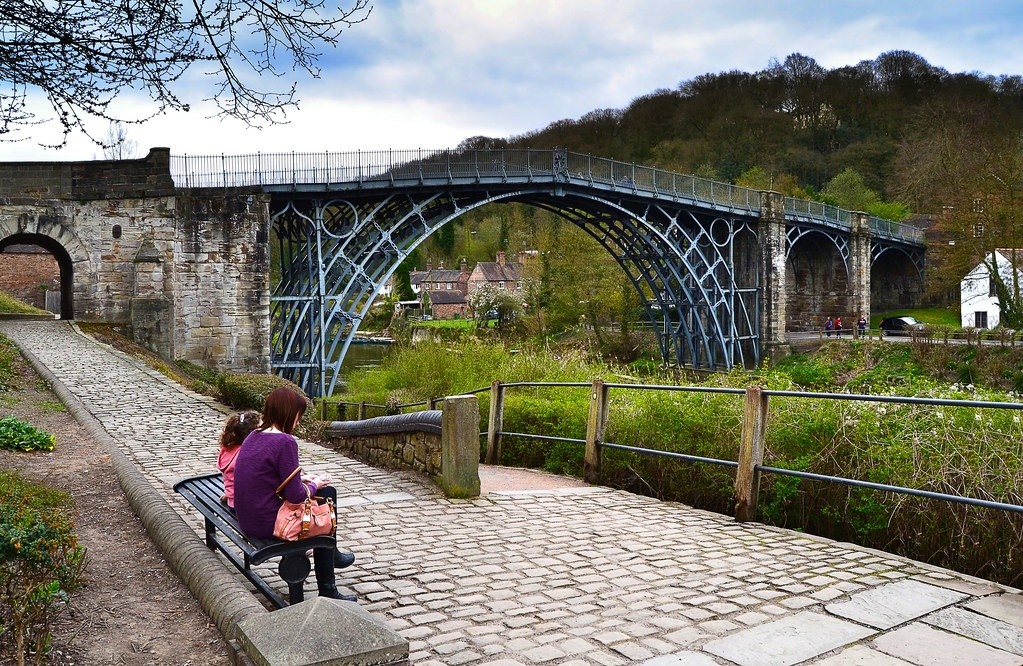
[464,205,469,264]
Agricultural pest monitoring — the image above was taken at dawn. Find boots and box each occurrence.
[317,576,357,602]
[334,547,355,568]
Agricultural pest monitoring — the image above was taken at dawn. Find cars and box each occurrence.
[421,315,433,321]
[879,314,931,339]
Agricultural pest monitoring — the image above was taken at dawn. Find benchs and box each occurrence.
[172,472,336,609]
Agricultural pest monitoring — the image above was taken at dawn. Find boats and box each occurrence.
[329,330,398,344]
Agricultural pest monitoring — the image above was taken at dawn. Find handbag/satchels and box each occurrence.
[274,466,337,542]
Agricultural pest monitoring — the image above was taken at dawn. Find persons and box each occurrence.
[216,410,262,516]
[834,318,842,338]
[857,316,866,337]
[824,316,833,337]
[234,388,357,602]
